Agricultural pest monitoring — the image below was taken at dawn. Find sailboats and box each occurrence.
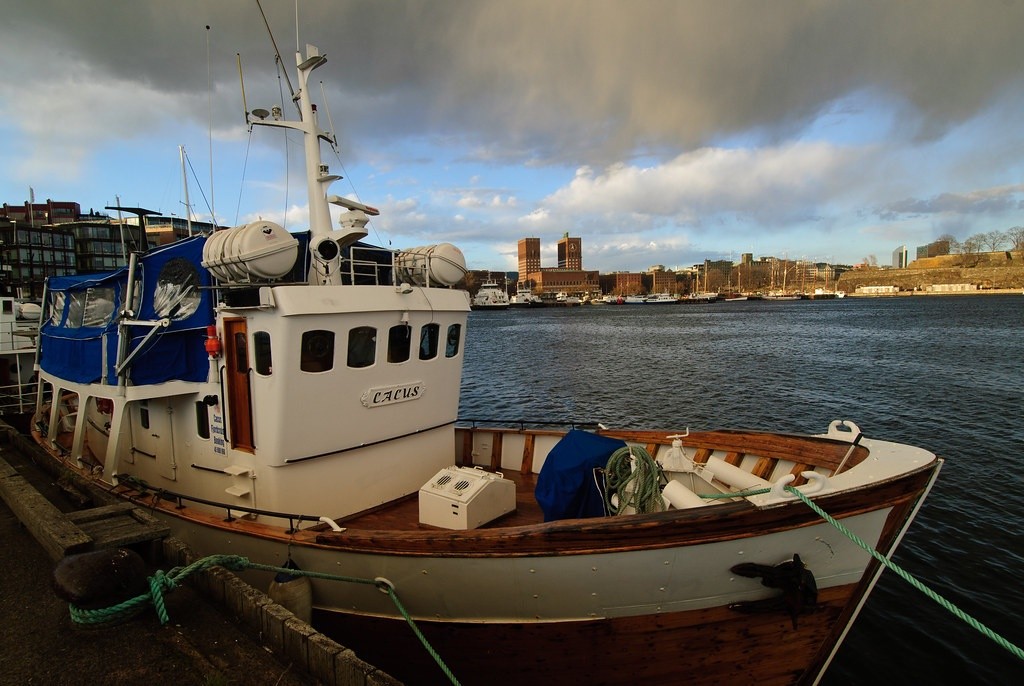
[31,0,946,685]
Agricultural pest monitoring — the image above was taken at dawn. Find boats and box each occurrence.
[471,269,847,312]
